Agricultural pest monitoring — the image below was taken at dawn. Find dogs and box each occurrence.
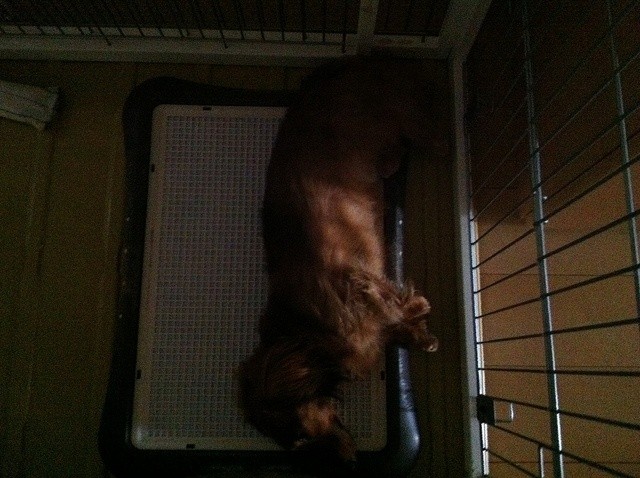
[242,57,450,464]
